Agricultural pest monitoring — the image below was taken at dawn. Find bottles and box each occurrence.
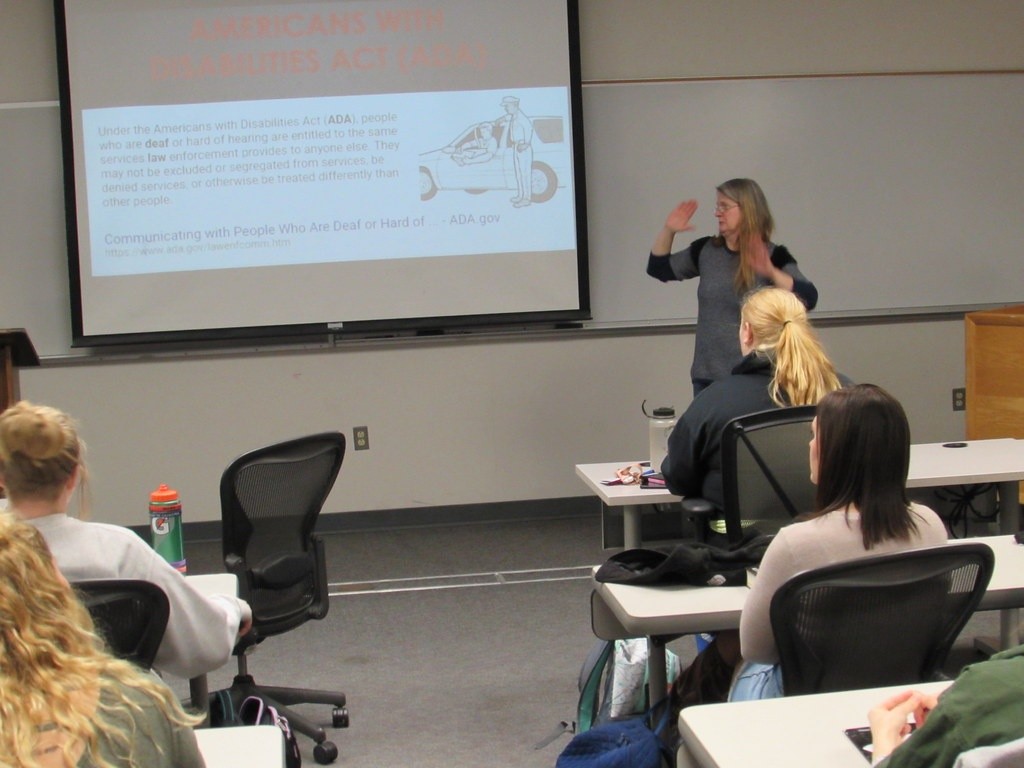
[641,400,679,474]
[147,484,187,578]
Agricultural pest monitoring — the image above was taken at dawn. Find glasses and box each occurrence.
[714,204,740,212]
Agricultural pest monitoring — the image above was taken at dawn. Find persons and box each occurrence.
[868,644,1024,768]
[647,179,817,396]
[661,286,852,546]
[0,398,252,678]
[727,383,950,702]
[0,508,208,768]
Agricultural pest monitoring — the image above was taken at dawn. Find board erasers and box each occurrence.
[553,323,584,328]
[415,330,445,336]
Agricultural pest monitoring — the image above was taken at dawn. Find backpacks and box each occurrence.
[556,691,671,768]
[209,688,302,768]
[576,635,684,734]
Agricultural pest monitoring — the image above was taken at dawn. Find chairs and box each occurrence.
[680,404,816,544]
[194,725,287,768]
[771,543,995,697]
[70,579,170,672]
[178,433,349,765]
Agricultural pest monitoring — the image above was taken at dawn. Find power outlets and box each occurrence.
[953,388,966,411]
[353,426,369,450]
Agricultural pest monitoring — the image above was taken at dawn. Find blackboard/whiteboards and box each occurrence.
[0,70,1024,365]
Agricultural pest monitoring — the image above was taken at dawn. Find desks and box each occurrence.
[576,438,1023,550]
[590,543,1024,768]
[673,681,955,768]
[184,572,238,727]
